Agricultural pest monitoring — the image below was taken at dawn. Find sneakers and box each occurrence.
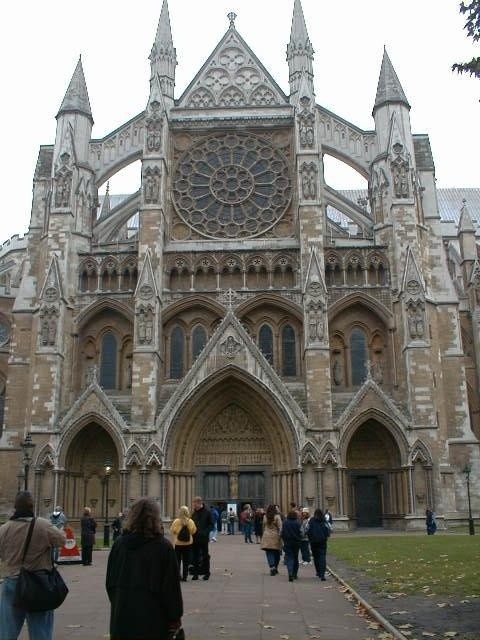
[299,559,313,567]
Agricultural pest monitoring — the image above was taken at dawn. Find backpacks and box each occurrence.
[176,517,191,542]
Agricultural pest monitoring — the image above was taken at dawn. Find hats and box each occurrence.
[302,506,311,513]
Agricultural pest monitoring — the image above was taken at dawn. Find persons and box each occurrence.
[111,512,124,541]
[50,505,67,560]
[0,491,67,640]
[170,496,333,582]
[80,507,97,566]
[426,506,434,535]
[105,498,183,640]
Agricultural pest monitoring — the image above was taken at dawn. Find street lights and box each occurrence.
[19,432,36,491]
[462,466,476,535]
[103,460,113,547]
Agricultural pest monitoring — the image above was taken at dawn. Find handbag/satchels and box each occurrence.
[13,565,70,612]
